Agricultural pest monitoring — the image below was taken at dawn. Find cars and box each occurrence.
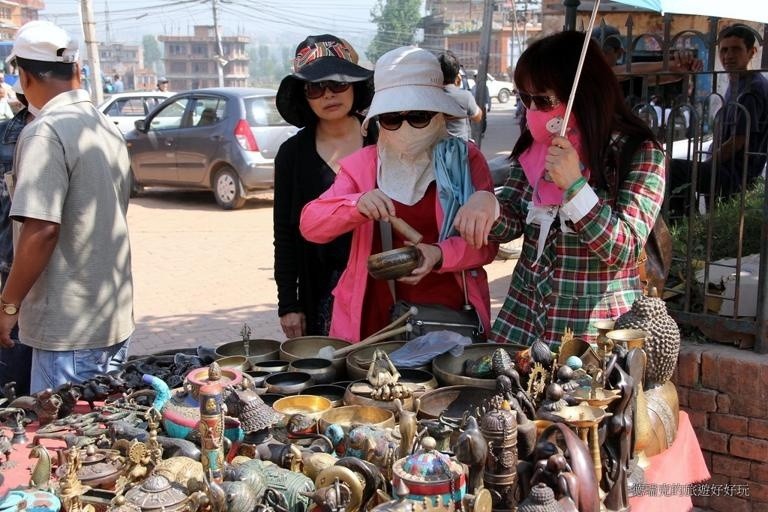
[124,90,302,210]
[456,71,515,103]
[97,90,182,135]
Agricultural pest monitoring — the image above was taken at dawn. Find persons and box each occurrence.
[1,20,136,394]
[661,23,768,226]
[455,32,664,353]
[82,64,90,77]
[272,34,380,349]
[472,74,491,138]
[152,77,170,94]
[104,73,124,93]
[1,77,36,398]
[436,49,482,141]
[300,46,499,345]
[1,72,26,122]
[590,25,704,114]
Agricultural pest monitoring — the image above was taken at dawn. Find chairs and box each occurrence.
[195,108,216,126]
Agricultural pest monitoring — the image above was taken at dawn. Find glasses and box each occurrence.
[379,111,431,130]
[518,92,560,112]
[303,80,350,99]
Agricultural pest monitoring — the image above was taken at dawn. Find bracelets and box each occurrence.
[564,176,588,200]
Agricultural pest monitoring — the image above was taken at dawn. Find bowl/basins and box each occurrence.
[290,358,337,380]
[214,337,278,362]
[348,340,414,379]
[366,244,419,280]
[266,372,314,396]
[211,353,248,372]
[300,385,346,404]
[344,381,415,419]
[416,385,499,418]
[274,396,334,420]
[388,367,439,392]
[252,358,287,372]
[320,405,396,434]
[244,369,270,386]
[432,344,540,389]
[279,334,353,363]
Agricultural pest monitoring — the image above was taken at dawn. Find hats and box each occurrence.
[276,34,375,128]
[4,20,79,63]
[157,77,169,83]
[361,47,468,136]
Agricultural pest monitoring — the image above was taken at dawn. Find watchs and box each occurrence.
[1,298,21,315]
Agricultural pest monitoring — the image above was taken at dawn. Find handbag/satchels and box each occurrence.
[621,132,673,299]
[390,302,484,345]
